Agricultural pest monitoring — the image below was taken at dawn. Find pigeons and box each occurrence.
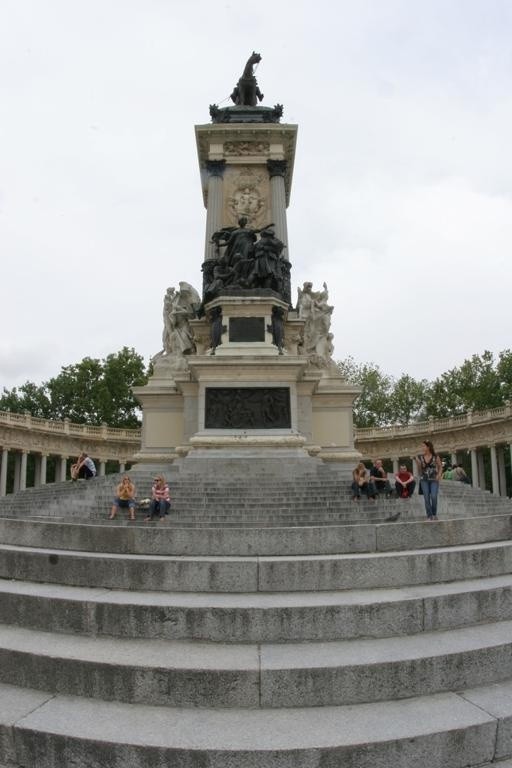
[384,511,401,523]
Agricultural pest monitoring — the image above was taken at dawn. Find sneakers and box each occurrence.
[143,516,165,522]
[427,514,438,521]
[353,492,412,501]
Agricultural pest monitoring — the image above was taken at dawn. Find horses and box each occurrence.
[237,51,262,107]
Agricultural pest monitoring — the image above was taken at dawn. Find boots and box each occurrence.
[129,507,136,521]
[108,504,119,520]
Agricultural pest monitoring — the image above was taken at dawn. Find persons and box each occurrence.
[369,459,393,498]
[71,455,84,481]
[450,465,457,481]
[452,463,471,485]
[110,476,137,520]
[351,462,377,502]
[161,217,335,368]
[414,439,442,521]
[392,463,415,499]
[142,473,171,522]
[71,451,97,483]
[440,466,453,480]
